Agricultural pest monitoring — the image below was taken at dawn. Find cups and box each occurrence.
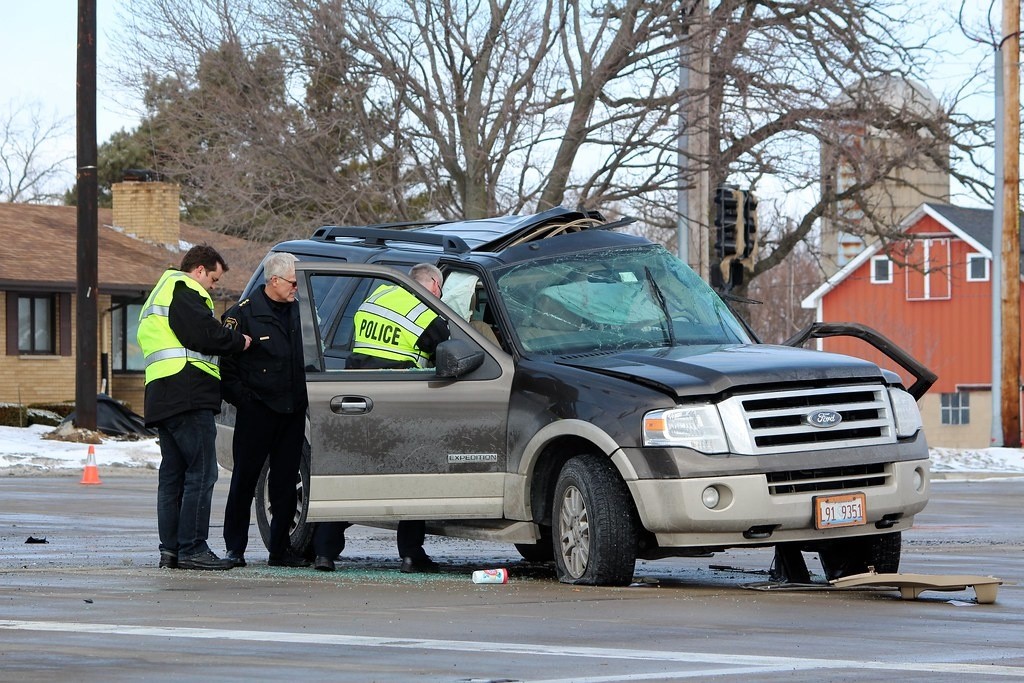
[471,567,508,584]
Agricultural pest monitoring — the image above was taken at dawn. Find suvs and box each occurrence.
[215,207,938,587]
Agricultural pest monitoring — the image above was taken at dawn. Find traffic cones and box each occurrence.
[80,446,102,484]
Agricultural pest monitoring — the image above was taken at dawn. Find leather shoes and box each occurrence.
[178,551,234,569]
[314,555,335,571]
[400,557,439,573]
[267,553,309,567]
[159,552,178,568]
[224,549,246,566]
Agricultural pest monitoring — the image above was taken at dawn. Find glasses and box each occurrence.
[270,275,297,288]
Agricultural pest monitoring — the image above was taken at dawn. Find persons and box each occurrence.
[218,252,310,568]
[137,240,253,571]
[314,263,450,575]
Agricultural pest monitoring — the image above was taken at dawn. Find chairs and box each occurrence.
[514,293,584,344]
